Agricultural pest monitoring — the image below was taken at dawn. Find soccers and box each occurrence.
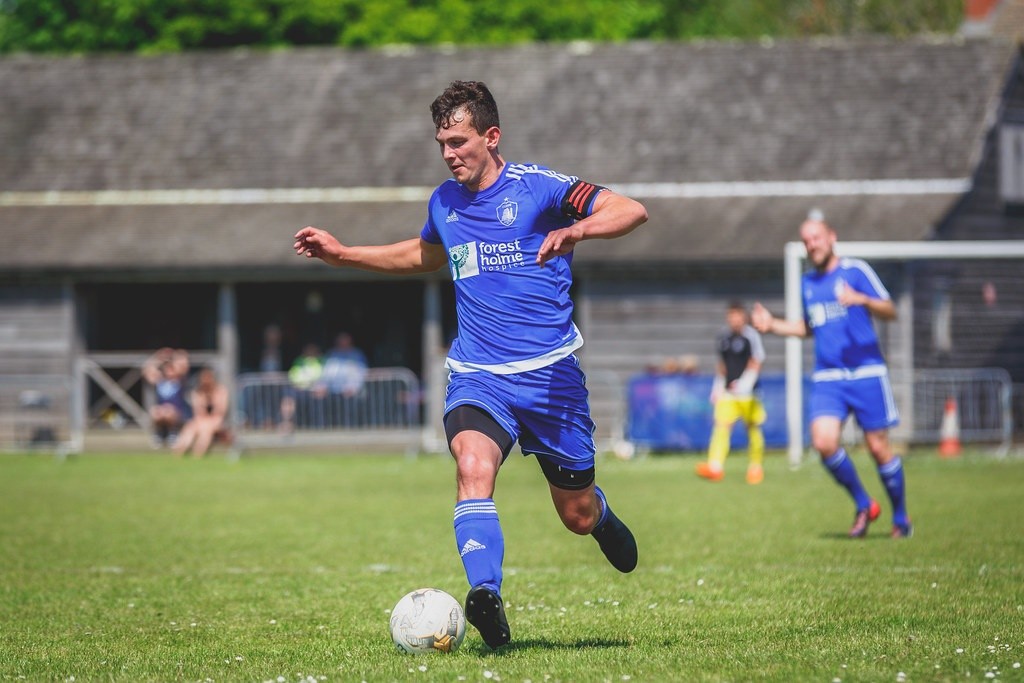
[389,587,467,657]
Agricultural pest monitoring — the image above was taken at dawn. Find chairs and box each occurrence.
[13,388,59,450]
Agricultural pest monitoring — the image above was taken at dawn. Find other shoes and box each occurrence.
[697,464,725,481]
[747,467,762,483]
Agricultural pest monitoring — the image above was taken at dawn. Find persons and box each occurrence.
[316,332,369,424]
[287,343,325,428]
[141,348,233,460]
[751,219,914,539]
[255,324,287,425]
[293,80,649,648]
[693,304,767,484]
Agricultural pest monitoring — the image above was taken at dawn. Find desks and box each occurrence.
[623,371,813,456]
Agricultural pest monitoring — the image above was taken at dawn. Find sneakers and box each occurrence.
[852,499,881,538]
[591,485,638,575]
[891,518,912,539]
[464,585,510,649]
